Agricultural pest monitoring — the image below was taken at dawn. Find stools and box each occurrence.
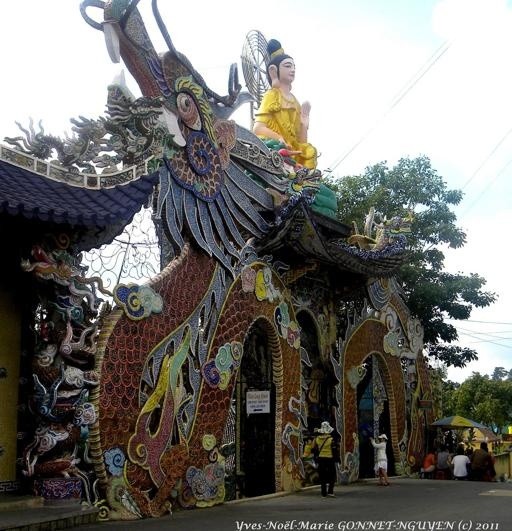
[437,471,449,480]
[420,472,432,479]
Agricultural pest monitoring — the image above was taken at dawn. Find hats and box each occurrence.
[377,433,389,441]
[316,421,335,435]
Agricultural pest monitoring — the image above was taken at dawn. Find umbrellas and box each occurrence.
[430,415,487,450]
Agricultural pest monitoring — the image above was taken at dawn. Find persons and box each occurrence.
[420,431,498,482]
[370,434,390,486]
[253,38,318,171]
[314,422,341,497]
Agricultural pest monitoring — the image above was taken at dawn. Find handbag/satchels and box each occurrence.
[312,446,321,463]
